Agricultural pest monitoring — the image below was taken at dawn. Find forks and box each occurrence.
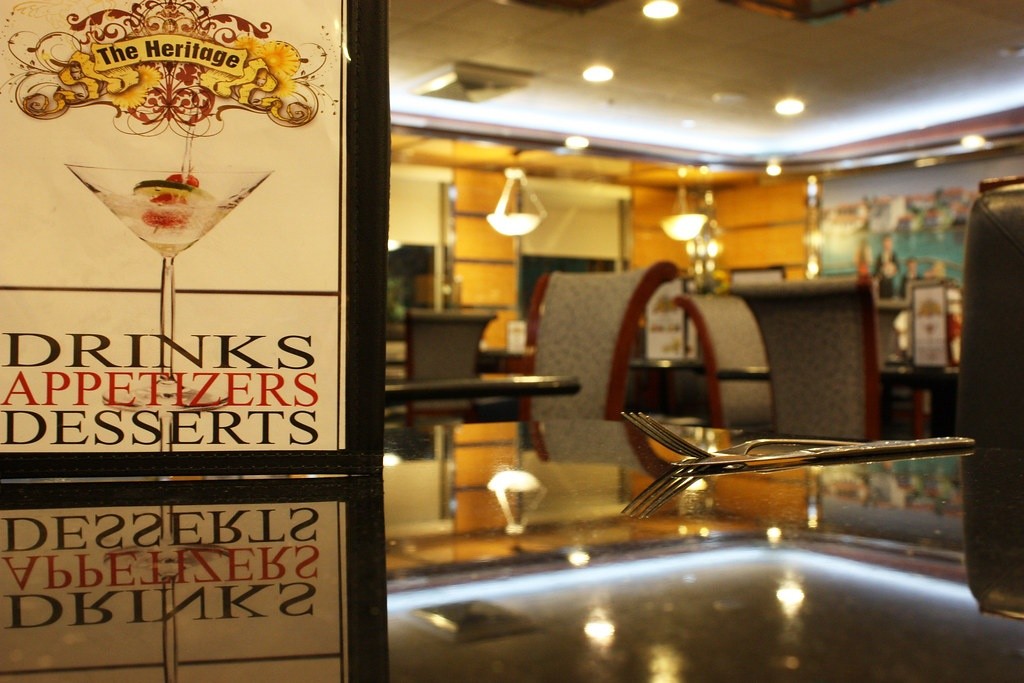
[622,410,867,459]
[622,456,976,520]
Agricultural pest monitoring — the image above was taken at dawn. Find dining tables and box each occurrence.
[381,416,1024,682]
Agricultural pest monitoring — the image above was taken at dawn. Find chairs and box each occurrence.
[387,258,883,443]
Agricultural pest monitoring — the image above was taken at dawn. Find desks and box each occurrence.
[717,362,959,383]
[386,375,580,405]
[626,358,707,374]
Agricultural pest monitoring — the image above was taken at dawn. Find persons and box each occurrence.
[852,231,924,302]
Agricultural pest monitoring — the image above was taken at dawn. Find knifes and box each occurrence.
[670,436,975,465]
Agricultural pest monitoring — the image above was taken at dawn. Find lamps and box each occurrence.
[659,185,709,241]
[486,167,546,236]
